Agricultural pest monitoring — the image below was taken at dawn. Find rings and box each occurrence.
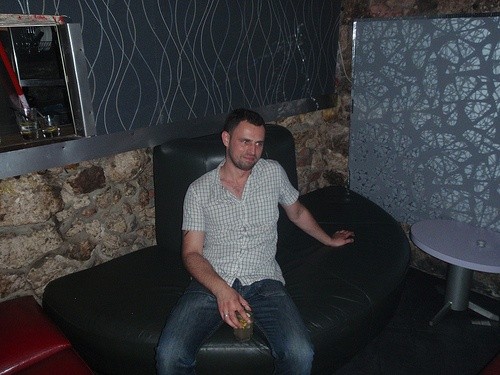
[223,314,229,317]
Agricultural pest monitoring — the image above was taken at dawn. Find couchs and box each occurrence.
[39,124,412,375]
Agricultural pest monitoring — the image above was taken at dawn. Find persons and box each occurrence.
[152,107,356,375]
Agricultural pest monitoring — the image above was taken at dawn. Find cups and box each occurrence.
[234,312,253,342]
[37,115,61,138]
[14,107,37,139]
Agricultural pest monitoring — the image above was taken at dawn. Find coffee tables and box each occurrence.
[408,218,500,329]
